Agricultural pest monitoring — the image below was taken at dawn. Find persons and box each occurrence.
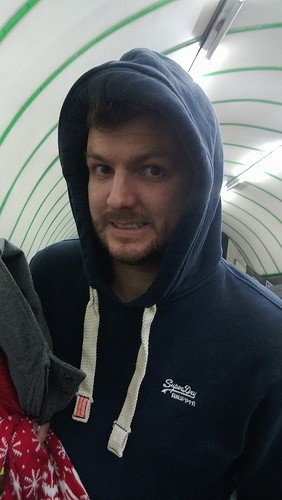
[27,46,282,500]
